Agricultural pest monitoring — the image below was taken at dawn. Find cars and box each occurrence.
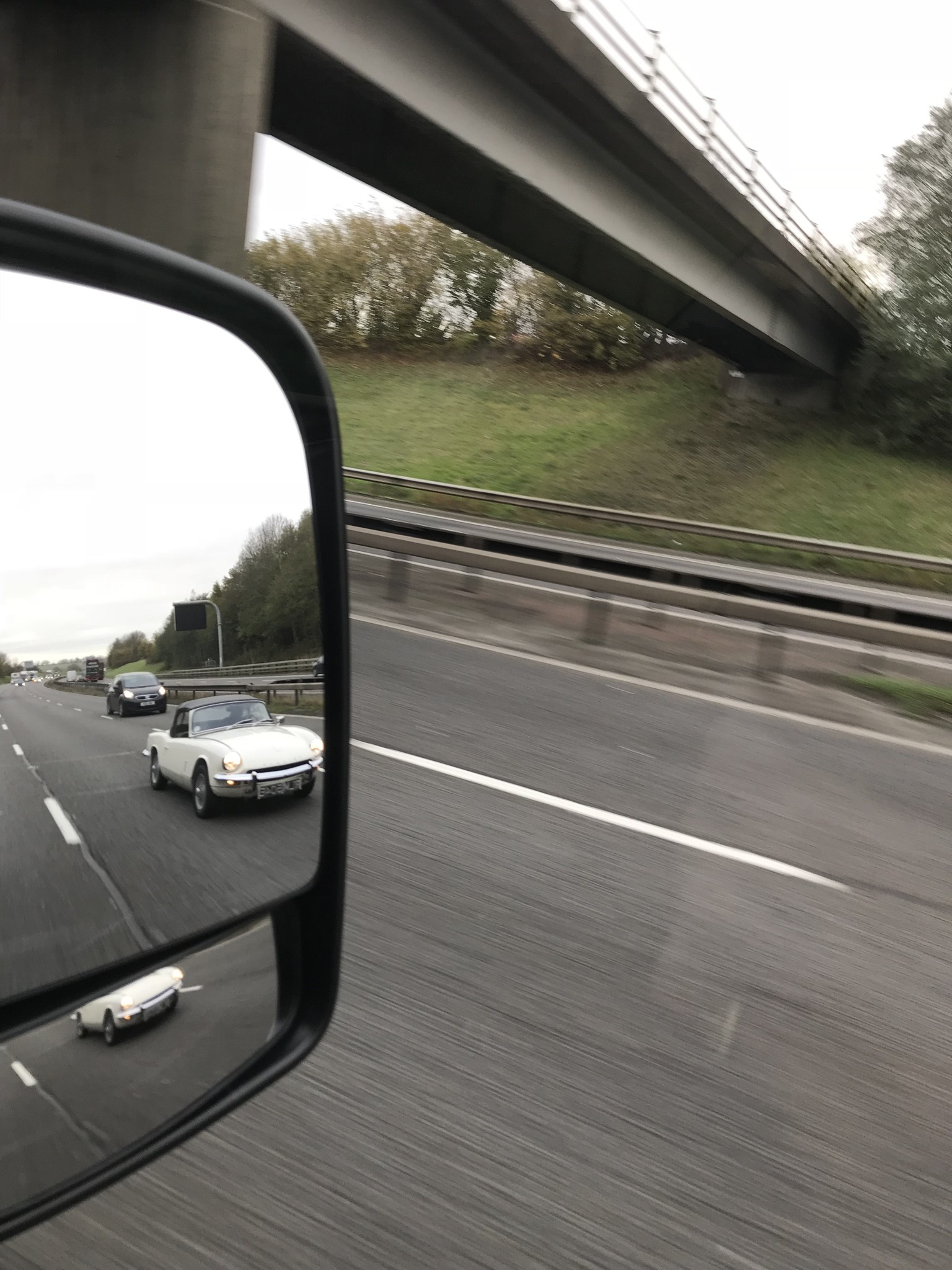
[71,965,184,1045]
[311,655,325,677]
[105,672,170,716]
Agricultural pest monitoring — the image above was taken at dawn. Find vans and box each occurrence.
[11,669,61,687]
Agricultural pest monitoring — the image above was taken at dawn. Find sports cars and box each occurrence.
[146,693,324,817]
